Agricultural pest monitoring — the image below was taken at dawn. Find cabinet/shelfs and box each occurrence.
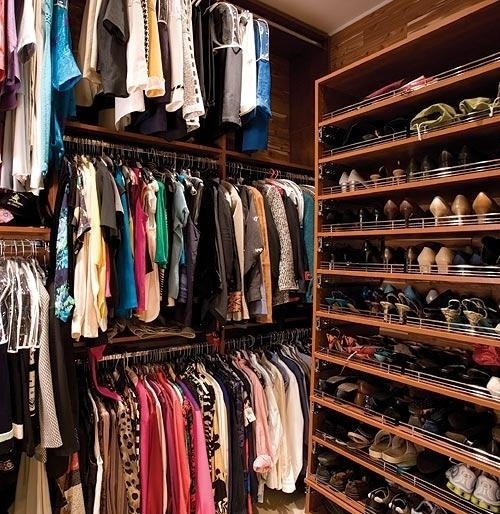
[66,0,322,348]
[311,57,500,510]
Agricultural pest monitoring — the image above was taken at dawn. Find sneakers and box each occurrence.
[312,431,499,514]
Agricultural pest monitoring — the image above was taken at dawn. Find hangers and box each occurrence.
[64,135,310,185]
[83,327,311,363]
[0,238,52,263]
